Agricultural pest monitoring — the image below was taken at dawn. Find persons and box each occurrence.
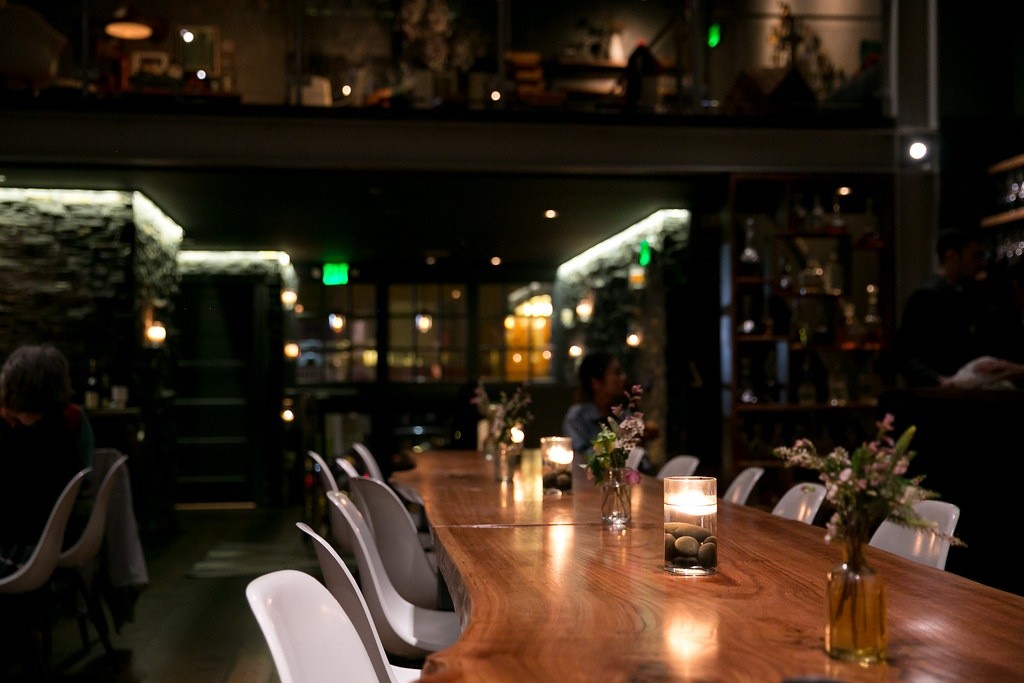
[562,350,662,477]
[0,343,95,566]
[878,223,1023,454]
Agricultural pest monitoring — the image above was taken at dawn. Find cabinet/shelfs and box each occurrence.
[719,172,894,492]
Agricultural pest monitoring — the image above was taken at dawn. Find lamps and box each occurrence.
[104,5,154,41]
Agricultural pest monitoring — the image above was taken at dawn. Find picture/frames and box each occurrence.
[176,22,221,81]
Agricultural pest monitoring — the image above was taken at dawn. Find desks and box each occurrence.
[391,448,1024,683]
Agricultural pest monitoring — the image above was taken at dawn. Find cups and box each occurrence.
[110,386,129,409]
[660,476,719,578]
[539,436,574,496]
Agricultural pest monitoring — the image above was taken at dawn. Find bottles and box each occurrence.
[797,362,816,406]
[771,250,881,351]
[83,358,100,410]
[825,362,848,407]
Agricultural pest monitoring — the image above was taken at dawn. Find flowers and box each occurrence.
[579,382,654,484]
[769,417,969,550]
[466,384,528,442]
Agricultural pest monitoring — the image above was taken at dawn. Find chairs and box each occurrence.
[505,49,570,118]
[868,500,960,571]
[772,483,828,525]
[625,446,646,470]
[656,453,699,483]
[0,450,149,683]
[104,50,135,92]
[723,467,765,506]
[246,441,460,683]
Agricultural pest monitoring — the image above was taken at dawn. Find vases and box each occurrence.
[483,430,497,462]
[497,427,521,481]
[827,532,888,666]
